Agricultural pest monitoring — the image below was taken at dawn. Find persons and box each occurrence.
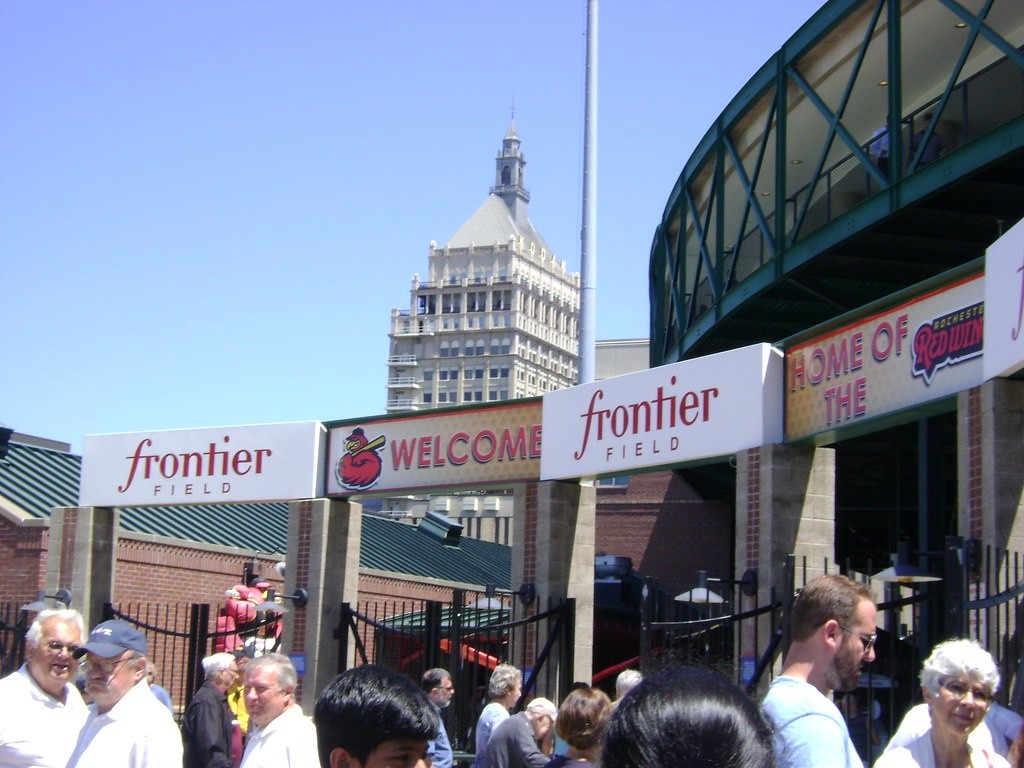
[472,651,774,768]
[315,666,455,768]
[0,608,184,768]
[760,575,1024,768]
[178,645,322,768]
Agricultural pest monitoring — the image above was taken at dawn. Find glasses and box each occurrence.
[943,682,994,713]
[813,621,878,652]
[37,641,77,654]
[78,657,135,672]
[438,686,452,692]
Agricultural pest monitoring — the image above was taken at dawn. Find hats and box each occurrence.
[72,619,147,660]
